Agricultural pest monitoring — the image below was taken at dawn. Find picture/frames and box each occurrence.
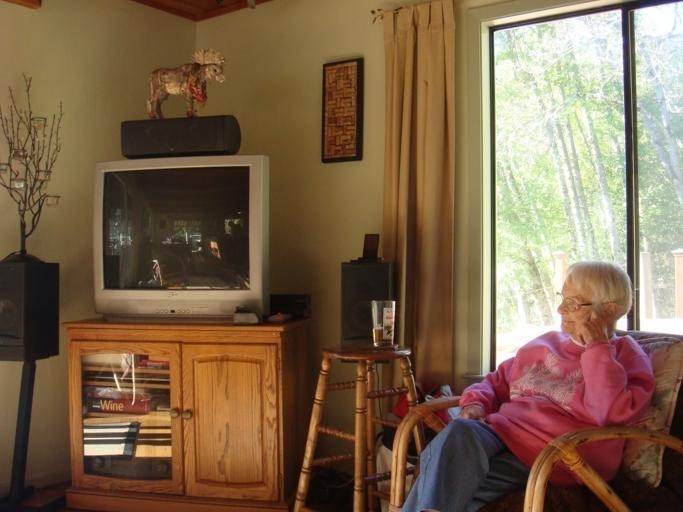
[320,57,364,163]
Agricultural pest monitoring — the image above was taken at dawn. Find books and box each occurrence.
[82,352,171,415]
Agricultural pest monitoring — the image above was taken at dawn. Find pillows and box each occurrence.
[615,328,683,489]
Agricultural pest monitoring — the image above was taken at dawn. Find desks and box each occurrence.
[293,346,427,512]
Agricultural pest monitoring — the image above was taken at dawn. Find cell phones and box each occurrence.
[362,232,380,258]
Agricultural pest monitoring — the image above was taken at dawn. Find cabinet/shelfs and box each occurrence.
[59,315,315,512]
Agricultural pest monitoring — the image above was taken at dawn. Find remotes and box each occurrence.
[269,306,302,324]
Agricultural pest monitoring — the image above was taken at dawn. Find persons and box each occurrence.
[399,260,657,512]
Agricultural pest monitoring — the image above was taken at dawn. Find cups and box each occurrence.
[371,300,396,347]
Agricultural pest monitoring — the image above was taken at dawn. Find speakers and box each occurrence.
[340,262,395,350]
[119,113,242,159]
[0,252,60,363]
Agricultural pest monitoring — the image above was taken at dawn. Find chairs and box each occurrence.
[386,322,683,511]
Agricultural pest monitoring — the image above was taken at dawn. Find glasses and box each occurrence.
[555,292,614,315]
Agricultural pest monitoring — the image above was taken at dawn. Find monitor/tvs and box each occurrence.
[92,154,272,323]
